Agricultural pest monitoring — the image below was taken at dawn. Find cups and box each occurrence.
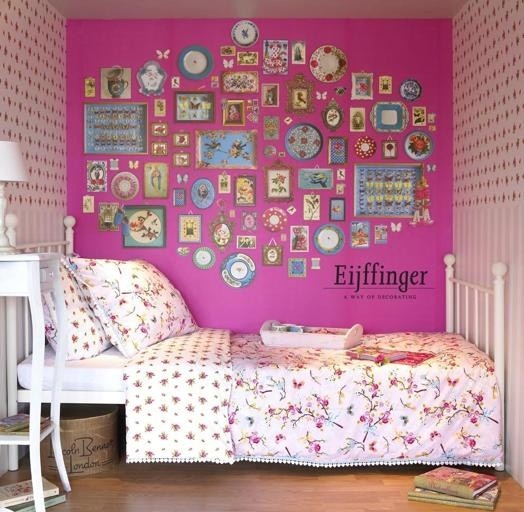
[289,326,304,333]
[271,325,287,332]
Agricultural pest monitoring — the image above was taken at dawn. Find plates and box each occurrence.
[107,19,433,290]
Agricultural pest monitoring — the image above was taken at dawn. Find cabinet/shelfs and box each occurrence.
[0,249,73,512]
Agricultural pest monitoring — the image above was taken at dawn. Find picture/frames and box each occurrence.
[74,19,435,292]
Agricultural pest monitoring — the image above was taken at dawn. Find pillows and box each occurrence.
[20,251,114,365]
[57,252,196,360]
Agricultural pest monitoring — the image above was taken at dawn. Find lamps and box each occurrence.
[0,139,28,256]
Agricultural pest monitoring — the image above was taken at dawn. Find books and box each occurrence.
[407,466,502,511]
[0,412,68,512]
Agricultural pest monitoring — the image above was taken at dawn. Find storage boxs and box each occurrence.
[22,401,123,483]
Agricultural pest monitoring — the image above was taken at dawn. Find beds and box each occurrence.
[0,210,512,484]
